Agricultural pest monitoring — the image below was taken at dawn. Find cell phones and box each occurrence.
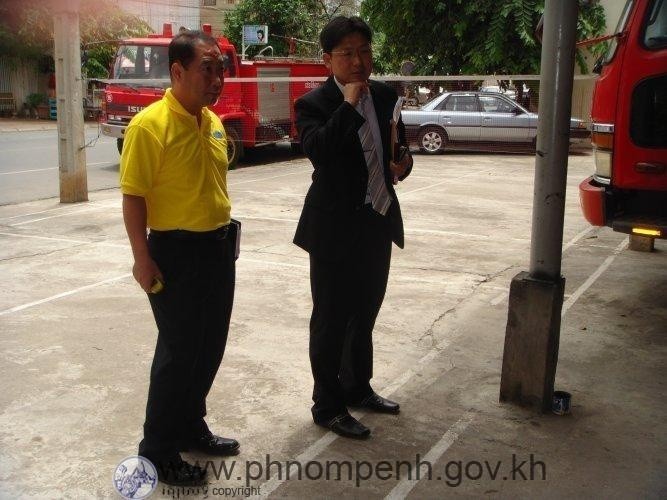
[150,277,165,295]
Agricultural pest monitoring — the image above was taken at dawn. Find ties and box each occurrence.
[358,95,394,217]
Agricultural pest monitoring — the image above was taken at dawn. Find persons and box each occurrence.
[120,29,239,489]
[294,16,414,440]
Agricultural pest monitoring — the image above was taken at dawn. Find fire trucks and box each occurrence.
[95,20,343,168]
[579,0,667,241]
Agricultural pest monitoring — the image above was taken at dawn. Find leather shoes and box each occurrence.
[344,388,399,411]
[314,408,371,437]
[138,461,207,485]
[178,428,240,454]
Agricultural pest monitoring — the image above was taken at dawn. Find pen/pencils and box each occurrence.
[395,147,407,165]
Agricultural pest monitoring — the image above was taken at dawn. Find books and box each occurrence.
[390,96,404,185]
[230,219,241,262]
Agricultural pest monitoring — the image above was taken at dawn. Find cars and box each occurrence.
[396,85,593,153]
